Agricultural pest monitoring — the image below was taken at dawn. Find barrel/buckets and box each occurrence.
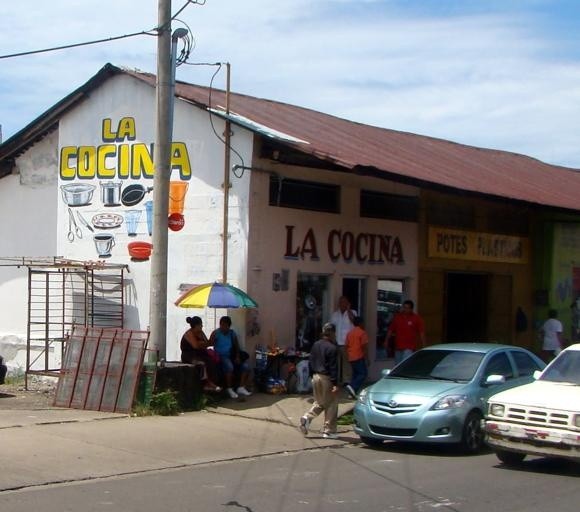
[167,179,190,214]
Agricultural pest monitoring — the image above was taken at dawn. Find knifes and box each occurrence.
[76,209,94,235]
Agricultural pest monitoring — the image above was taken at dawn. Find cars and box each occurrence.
[349,342,548,452]
[480,343,580,466]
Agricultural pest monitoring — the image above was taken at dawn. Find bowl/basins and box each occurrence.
[127,241,152,260]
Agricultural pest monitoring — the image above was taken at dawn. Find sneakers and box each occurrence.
[346,385,355,396]
[227,387,238,399]
[322,432,339,439]
[300,416,309,434]
[237,387,253,396]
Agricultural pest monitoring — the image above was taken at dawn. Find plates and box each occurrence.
[91,213,124,229]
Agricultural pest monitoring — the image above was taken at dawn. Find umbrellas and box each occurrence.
[174,282,259,332]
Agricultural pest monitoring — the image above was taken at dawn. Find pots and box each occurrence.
[59,179,154,207]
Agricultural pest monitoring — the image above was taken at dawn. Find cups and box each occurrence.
[93,232,114,258]
[123,208,142,237]
[144,199,152,238]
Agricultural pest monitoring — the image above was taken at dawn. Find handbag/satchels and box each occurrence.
[230,345,249,367]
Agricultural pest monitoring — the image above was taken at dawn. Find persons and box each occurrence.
[537,308,569,363]
[384,300,426,376]
[345,317,369,400]
[179,315,223,393]
[300,323,340,440]
[209,316,253,399]
[327,295,357,387]
